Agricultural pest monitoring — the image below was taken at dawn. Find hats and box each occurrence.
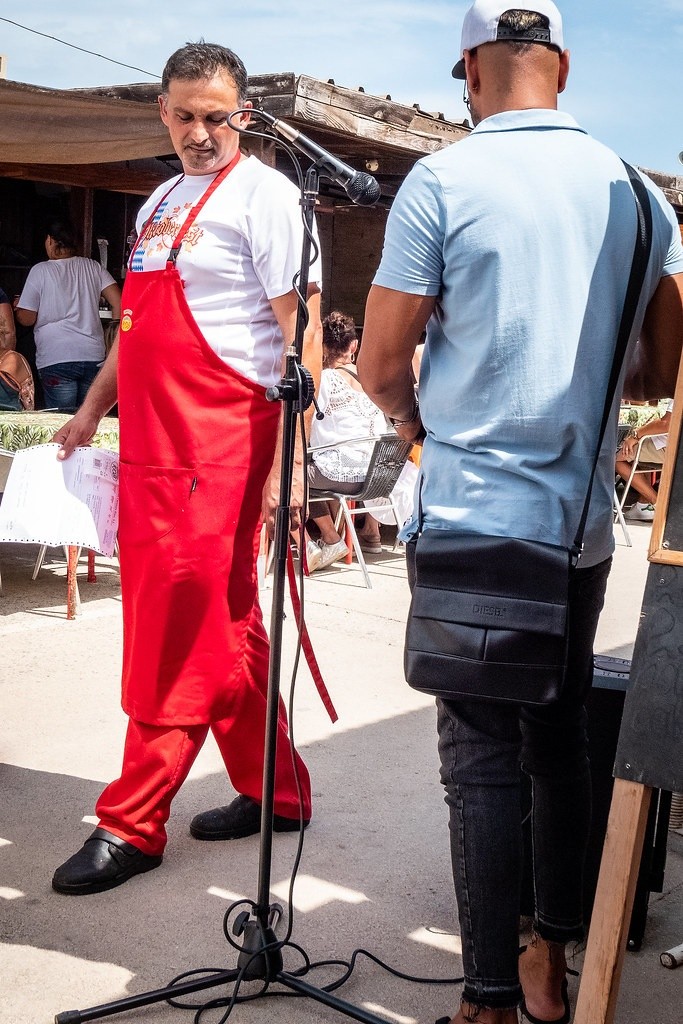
[451,0,563,79]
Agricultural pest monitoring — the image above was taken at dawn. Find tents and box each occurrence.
[0,78,276,260]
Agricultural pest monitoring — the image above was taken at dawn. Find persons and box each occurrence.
[49,42,324,897]
[0,215,121,412]
[282,0,683,1023]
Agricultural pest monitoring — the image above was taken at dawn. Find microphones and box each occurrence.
[260,111,382,208]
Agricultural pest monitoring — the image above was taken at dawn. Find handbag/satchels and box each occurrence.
[402,528,580,709]
[0,350,34,411]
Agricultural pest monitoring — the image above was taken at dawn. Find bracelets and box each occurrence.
[384,392,419,428]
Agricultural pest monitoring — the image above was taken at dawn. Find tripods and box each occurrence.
[54,154,393,1024]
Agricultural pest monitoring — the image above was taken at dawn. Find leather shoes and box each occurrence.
[52,828,163,894]
[189,792,311,840]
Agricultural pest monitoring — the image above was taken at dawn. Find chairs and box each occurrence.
[614,431,669,523]
[266,433,414,589]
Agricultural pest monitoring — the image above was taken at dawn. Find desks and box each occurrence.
[0,410,120,620]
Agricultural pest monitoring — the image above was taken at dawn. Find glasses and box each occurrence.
[463,73,470,113]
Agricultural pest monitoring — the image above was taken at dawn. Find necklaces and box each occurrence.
[336,362,353,367]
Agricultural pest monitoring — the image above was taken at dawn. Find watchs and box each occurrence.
[632,430,641,441]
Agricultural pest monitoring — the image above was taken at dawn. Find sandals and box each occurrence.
[517,945,579,1024]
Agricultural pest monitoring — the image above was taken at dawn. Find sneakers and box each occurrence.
[315,538,350,570]
[624,502,654,520]
[358,534,383,553]
[298,541,322,575]
